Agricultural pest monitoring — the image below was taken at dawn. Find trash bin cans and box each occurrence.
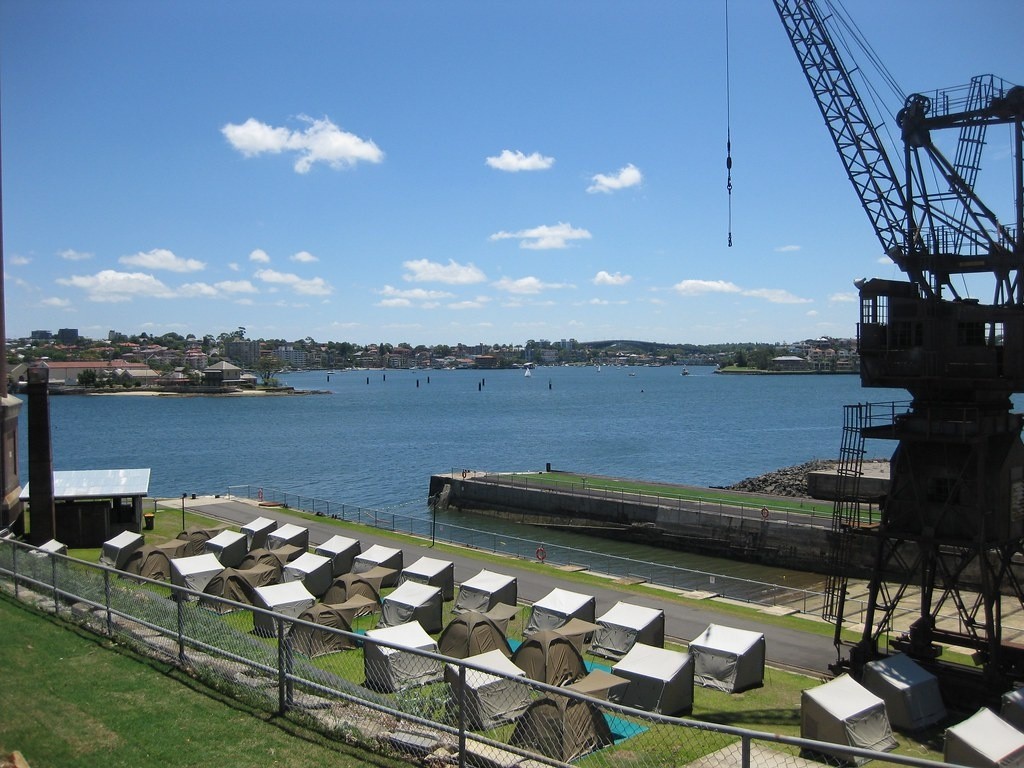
[144,513,155,530]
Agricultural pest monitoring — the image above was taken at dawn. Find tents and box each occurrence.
[283,593,376,657]
[397,556,454,601]
[252,580,317,636]
[445,648,533,730]
[801,671,900,767]
[350,543,403,587]
[943,706,1024,768]
[511,617,604,693]
[688,622,766,693]
[100,515,362,615]
[319,565,398,613]
[450,568,518,620]
[611,641,695,721]
[586,600,665,660]
[521,587,595,643]
[364,619,444,693]
[438,602,525,659]
[508,669,631,762]
[373,579,442,634]
[861,652,948,732]
